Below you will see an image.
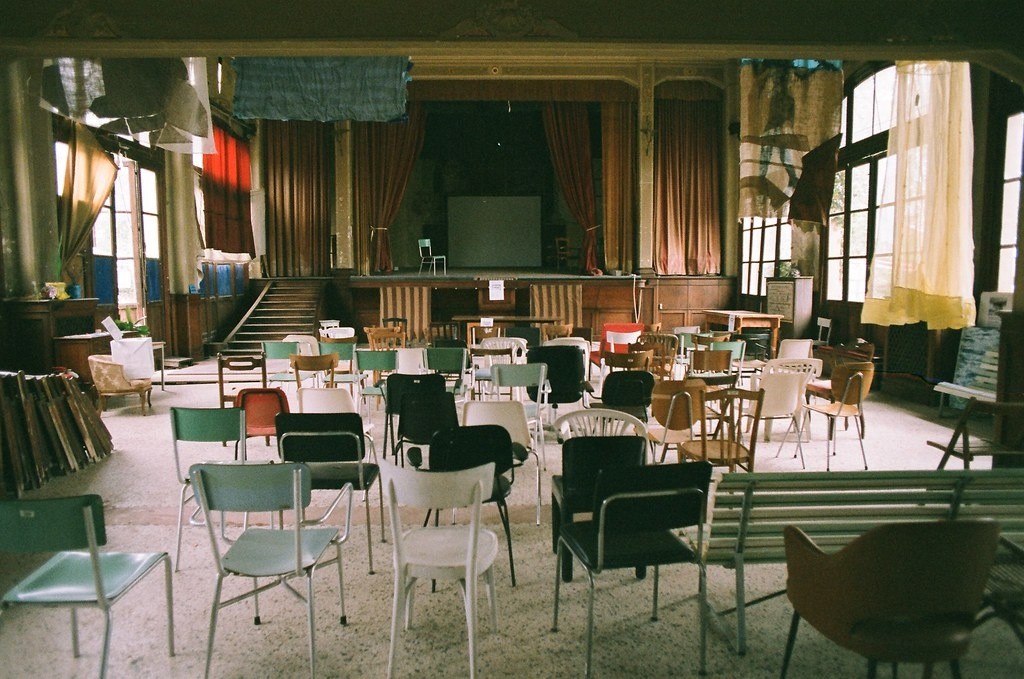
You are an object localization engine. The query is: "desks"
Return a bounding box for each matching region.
[702,309,785,361]
[53,331,140,409]
[149,341,166,392]
[14,299,99,375]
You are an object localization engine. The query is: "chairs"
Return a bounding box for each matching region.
[779,520,1000,678]
[169,407,251,572]
[914,396,1024,508]
[0,493,175,679]
[378,460,498,679]
[676,386,767,510]
[555,238,570,270]
[550,435,655,582]
[217,316,891,586]
[274,413,387,575]
[418,424,516,589]
[88,353,153,416]
[188,462,351,679]
[549,461,718,679]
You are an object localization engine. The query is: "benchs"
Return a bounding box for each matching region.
[702,469,1023,658]
[934,349,1000,428]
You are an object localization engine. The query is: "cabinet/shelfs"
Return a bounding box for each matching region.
[417,239,447,276]
[764,275,815,339]
